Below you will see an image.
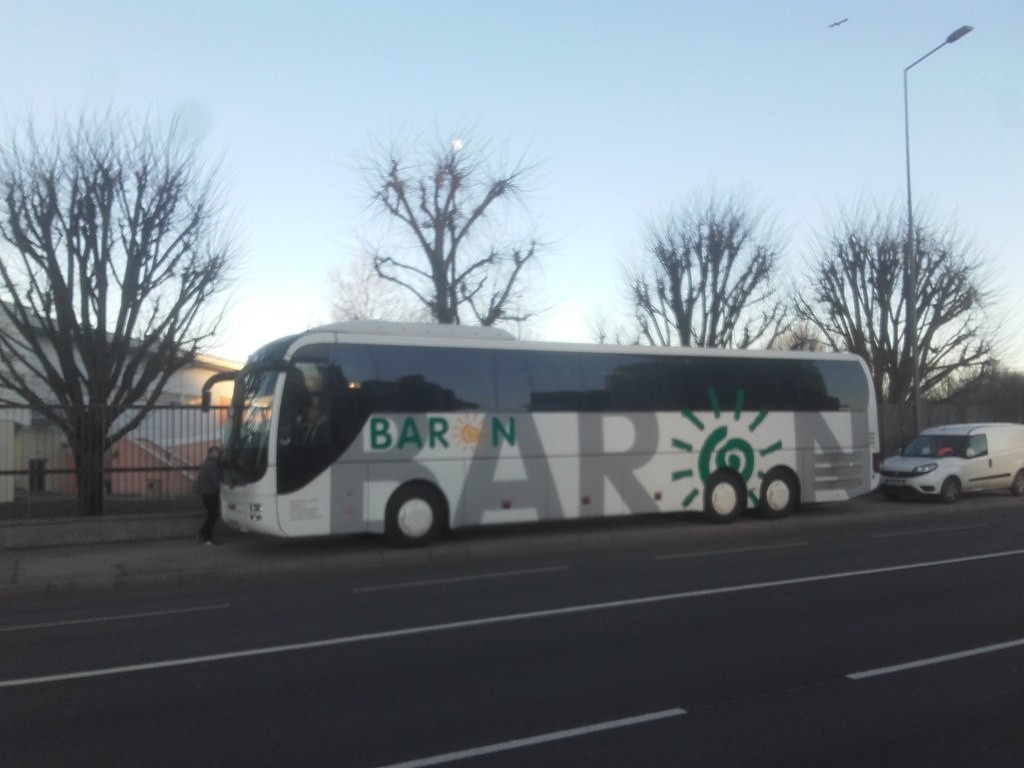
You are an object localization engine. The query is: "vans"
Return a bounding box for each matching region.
[875,420,1023,502]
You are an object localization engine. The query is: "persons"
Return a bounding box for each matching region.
[197,446,223,546]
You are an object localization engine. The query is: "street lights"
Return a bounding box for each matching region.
[901,25,975,438]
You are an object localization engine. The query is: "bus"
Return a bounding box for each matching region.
[201,318,886,551]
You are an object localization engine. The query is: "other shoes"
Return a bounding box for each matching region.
[198,538,220,546]
